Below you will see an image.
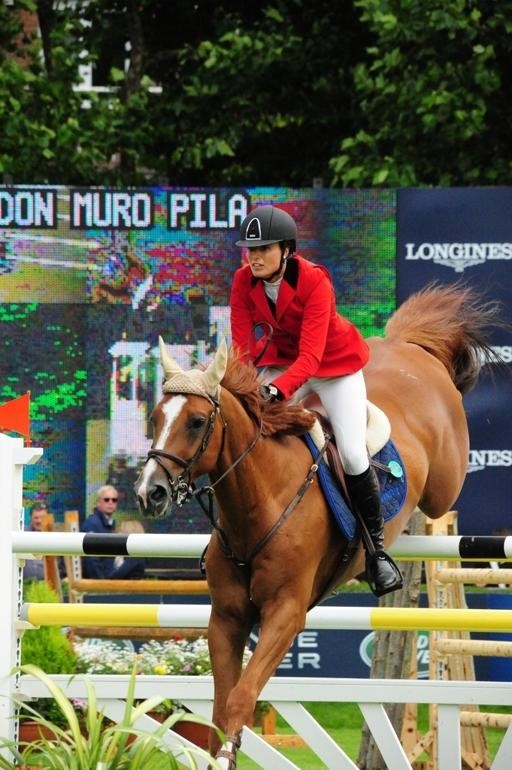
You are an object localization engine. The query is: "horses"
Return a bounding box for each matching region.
[132,272,512,769]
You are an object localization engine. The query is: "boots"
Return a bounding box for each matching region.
[344,467,398,593]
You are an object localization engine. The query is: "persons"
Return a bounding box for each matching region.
[229,205,403,597]
[79,484,124,579]
[23,502,67,581]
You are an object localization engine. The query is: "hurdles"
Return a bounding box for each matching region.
[13,528,511,767]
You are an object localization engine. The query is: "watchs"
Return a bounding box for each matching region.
[269,385,278,397]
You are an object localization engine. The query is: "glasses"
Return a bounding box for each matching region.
[104,498,118,502]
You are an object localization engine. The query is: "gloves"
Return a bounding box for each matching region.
[259,383,280,406]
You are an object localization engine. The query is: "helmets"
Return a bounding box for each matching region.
[235,205,296,248]
